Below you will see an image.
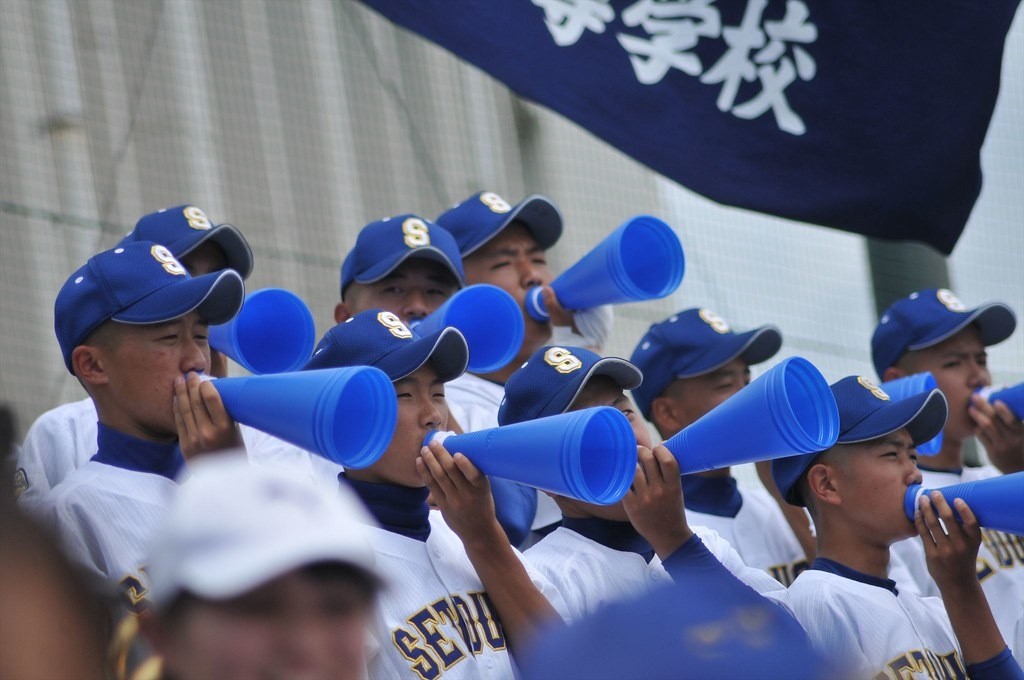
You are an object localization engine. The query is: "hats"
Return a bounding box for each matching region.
[630,308,783,421]
[770,375,949,507]
[498,345,643,427]
[872,287,1017,384]
[302,308,469,384]
[528,580,826,680]
[435,191,562,260]
[340,213,467,303]
[53,240,244,377]
[145,460,398,607]
[117,204,253,280]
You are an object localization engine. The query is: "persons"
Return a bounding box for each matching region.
[0,189,1023,680]
